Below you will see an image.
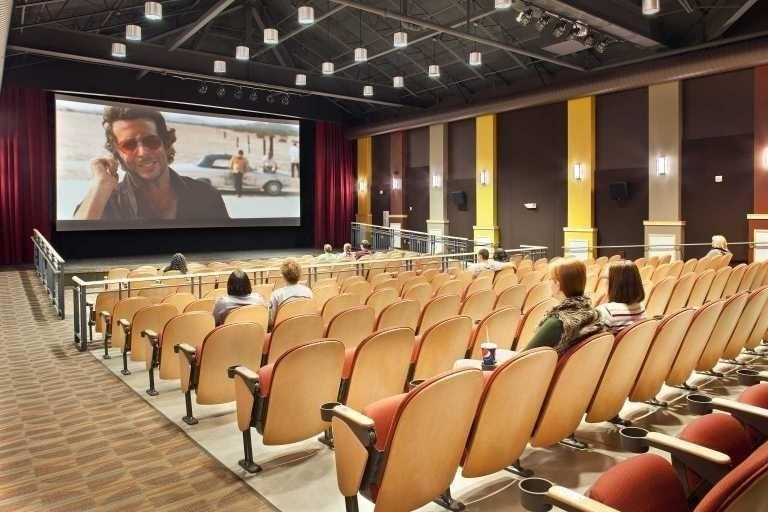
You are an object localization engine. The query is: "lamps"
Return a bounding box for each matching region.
[641,0,659,15]
[495,0,512,8]
[515,0,624,56]
[213,0,482,96]
[110,0,164,57]
[198,79,293,106]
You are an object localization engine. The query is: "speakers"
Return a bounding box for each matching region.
[609,182,628,202]
[451,191,466,205]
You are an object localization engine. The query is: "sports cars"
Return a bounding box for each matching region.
[168,153,289,194]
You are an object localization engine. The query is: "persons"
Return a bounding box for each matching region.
[356,239,372,259]
[268,257,314,328]
[464,248,494,277]
[705,234,730,258]
[213,268,269,326]
[74,105,230,220]
[316,244,338,263]
[261,156,278,174]
[594,259,647,336]
[453,257,605,371]
[491,247,517,273]
[288,142,300,177]
[338,242,354,258]
[230,150,246,197]
[163,252,188,274]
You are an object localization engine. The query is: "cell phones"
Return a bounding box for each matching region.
[109,150,120,177]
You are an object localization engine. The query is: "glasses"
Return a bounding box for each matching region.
[117,135,161,153]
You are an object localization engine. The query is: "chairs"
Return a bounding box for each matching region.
[227,339,344,470]
[618,412,767,498]
[316,367,483,512]
[319,327,417,449]
[172,322,265,424]
[517,442,766,511]
[96,250,768,387]
[685,380,767,422]
[584,316,661,430]
[409,346,561,479]
[523,332,613,451]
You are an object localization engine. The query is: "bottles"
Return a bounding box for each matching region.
[480,343,497,366]
